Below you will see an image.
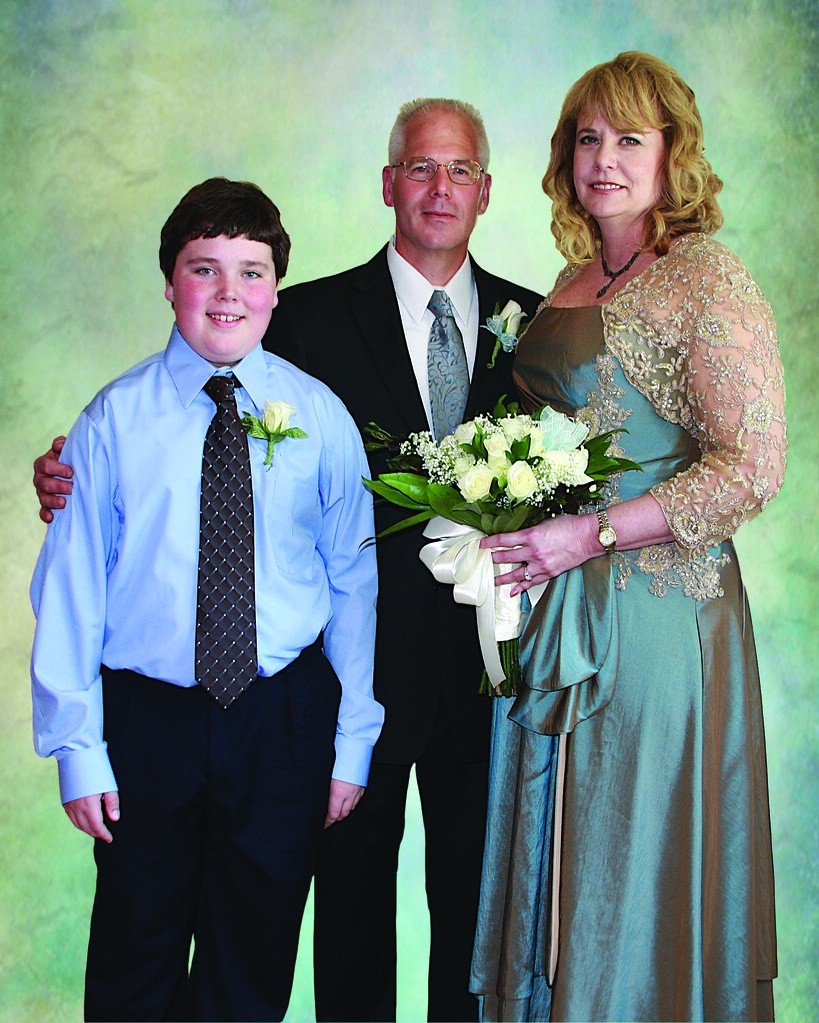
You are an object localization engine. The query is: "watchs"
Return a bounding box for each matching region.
[597,509,617,555]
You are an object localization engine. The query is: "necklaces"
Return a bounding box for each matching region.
[596,241,641,299]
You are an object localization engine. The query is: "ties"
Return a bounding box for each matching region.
[427,291,470,447]
[196,374,258,711]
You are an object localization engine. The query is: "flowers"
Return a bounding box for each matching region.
[356,393,644,699]
[240,400,308,465]
[480,300,528,369]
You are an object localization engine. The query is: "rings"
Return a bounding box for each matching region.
[523,565,533,581]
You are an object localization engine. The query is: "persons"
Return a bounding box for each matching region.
[32,97,546,1023]
[29,176,385,1023]
[466,50,790,1023]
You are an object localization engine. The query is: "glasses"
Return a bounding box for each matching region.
[390,157,485,186]
